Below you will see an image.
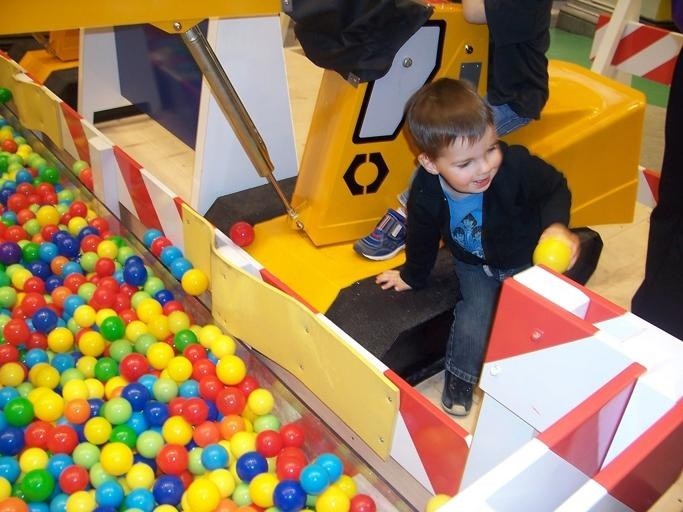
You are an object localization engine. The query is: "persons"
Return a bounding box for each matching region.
[374,78,580,417]
[352,1,550,260]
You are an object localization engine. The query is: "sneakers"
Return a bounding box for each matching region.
[354,209,408,260]
[442,369,473,415]
